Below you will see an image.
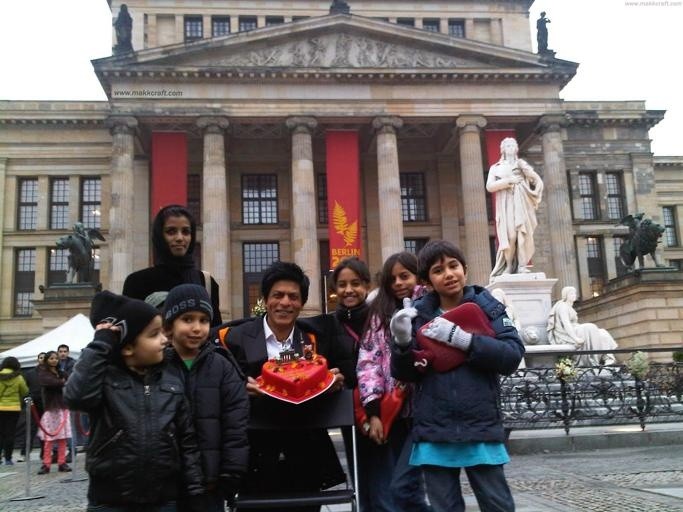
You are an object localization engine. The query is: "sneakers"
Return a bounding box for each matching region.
[16,454,25,461]
[37,465,48,474]
[66,452,70,462]
[58,463,71,471]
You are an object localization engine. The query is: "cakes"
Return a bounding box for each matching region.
[256,344,334,403]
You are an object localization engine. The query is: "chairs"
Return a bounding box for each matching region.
[222,384,359,512]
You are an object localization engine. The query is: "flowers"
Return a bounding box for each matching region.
[552,355,579,384]
[623,350,650,378]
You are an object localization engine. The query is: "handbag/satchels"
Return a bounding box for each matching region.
[418,301,496,373]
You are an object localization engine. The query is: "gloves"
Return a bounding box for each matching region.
[389,297,418,348]
[421,316,473,352]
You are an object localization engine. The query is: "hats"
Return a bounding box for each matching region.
[161,283,214,329]
[90,290,161,362]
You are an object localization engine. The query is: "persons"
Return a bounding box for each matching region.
[352,250,427,511]
[120,203,223,347]
[484,135,543,276]
[490,287,539,378]
[111,3,132,52]
[546,285,619,373]
[536,11,551,54]
[159,283,251,511]
[0,344,78,475]
[206,260,351,511]
[388,238,525,512]
[59,289,206,511]
[305,255,374,512]
